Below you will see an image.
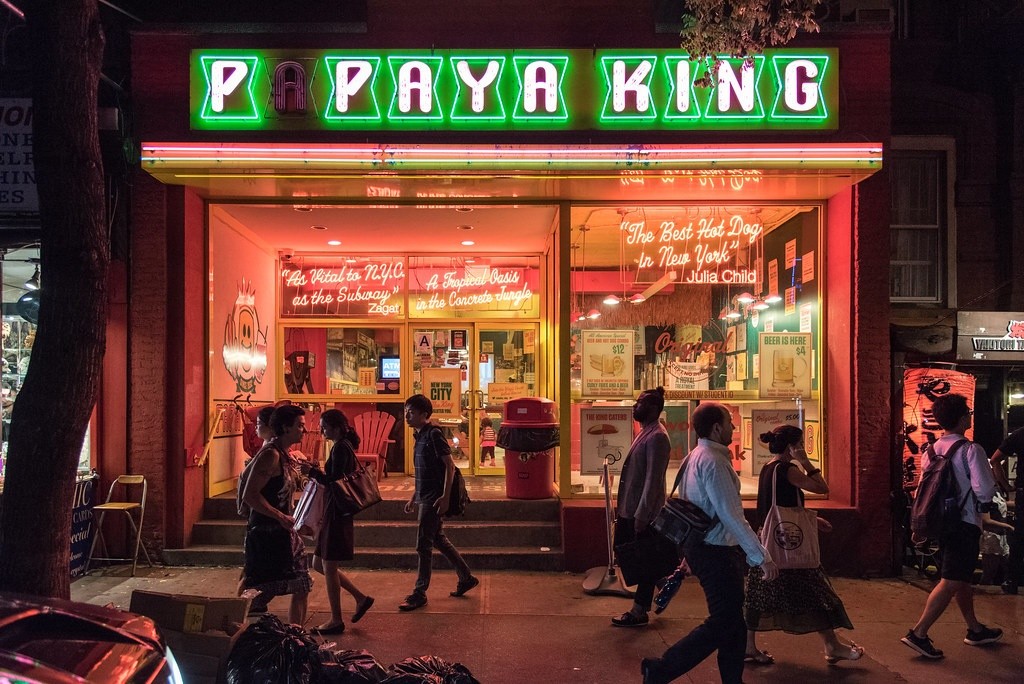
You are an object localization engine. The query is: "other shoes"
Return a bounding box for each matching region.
[351,597,374,623]
[309,623,345,634]
[1001,580,1018,595]
[490,463,496,466]
[480,463,483,466]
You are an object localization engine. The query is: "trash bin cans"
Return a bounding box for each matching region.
[501,397,560,500]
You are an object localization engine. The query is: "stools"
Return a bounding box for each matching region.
[84,475,154,578]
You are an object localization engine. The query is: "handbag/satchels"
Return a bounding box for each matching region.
[332,441,382,515]
[430,427,471,516]
[761,464,821,568]
[648,456,719,561]
[238,444,269,519]
[291,478,330,541]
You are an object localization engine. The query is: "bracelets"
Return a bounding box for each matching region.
[807,468,822,478]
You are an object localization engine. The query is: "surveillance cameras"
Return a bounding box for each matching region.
[284,248,294,260]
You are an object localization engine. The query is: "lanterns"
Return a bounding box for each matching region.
[902,366,975,497]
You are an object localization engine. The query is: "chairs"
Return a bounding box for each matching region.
[348,412,397,481]
[288,411,322,461]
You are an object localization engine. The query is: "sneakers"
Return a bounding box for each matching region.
[900,629,944,658]
[654,601,669,615]
[399,592,428,611]
[964,624,1003,646]
[641,657,659,684]
[450,574,479,597]
[611,612,649,627]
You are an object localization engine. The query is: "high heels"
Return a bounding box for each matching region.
[744,649,774,666]
[825,642,865,664]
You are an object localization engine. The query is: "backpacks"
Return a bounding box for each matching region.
[910,439,973,540]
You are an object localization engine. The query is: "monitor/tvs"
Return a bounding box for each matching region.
[378,355,400,382]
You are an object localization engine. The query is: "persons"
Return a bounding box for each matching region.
[237,405,313,628]
[991,425,1024,592]
[980,457,1015,558]
[461,401,496,466]
[638,402,779,684]
[611,387,690,627]
[744,423,865,664]
[900,393,1004,657]
[297,409,376,634]
[398,393,480,611]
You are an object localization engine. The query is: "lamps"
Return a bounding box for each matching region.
[572,226,677,322]
[717,211,781,320]
